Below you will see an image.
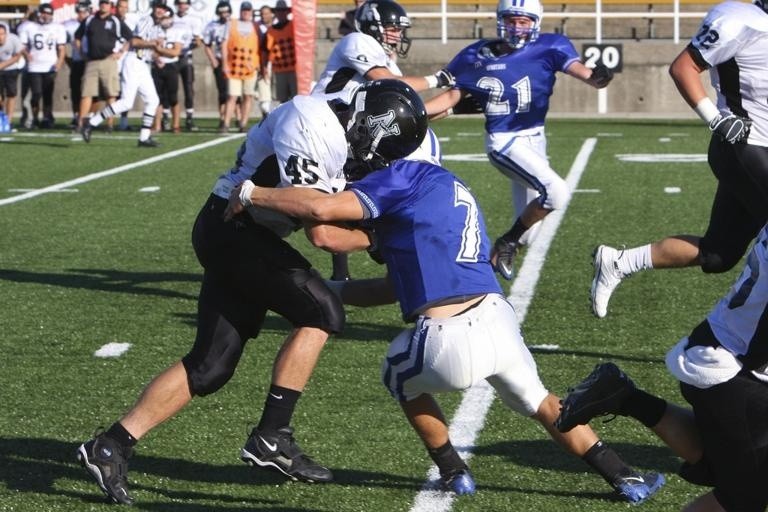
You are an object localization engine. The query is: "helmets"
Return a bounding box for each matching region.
[493,0,544,50]
[75,2,90,13]
[331,77,427,181]
[216,1,231,15]
[354,0,413,59]
[36,2,53,23]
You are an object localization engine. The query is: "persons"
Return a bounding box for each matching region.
[423,0,614,284]
[223,126,665,505]
[590,0,766,321]
[308,0,484,282]
[337,1,366,37]
[551,227,766,511]
[73,77,431,510]
[1,0,300,147]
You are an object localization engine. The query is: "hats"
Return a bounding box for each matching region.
[240,1,251,10]
[268,0,291,15]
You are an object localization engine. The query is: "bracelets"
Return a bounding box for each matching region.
[695,96,720,125]
[425,74,439,90]
[240,178,255,207]
[323,279,349,306]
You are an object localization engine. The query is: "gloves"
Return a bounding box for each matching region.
[434,67,457,90]
[708,110,752,146]
[452,94,482,114]
[589,63,615,88]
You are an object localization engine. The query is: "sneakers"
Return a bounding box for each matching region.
[436,469,474,495]
[185,118,198,132]
[493,235,516,282]
[615,473,665,507]
[74,425,135,506]
[81,117,92,143]
[553,362,634,435]
[588,243,625,318]
[237,421,333,484]
[138,136,161,146]
